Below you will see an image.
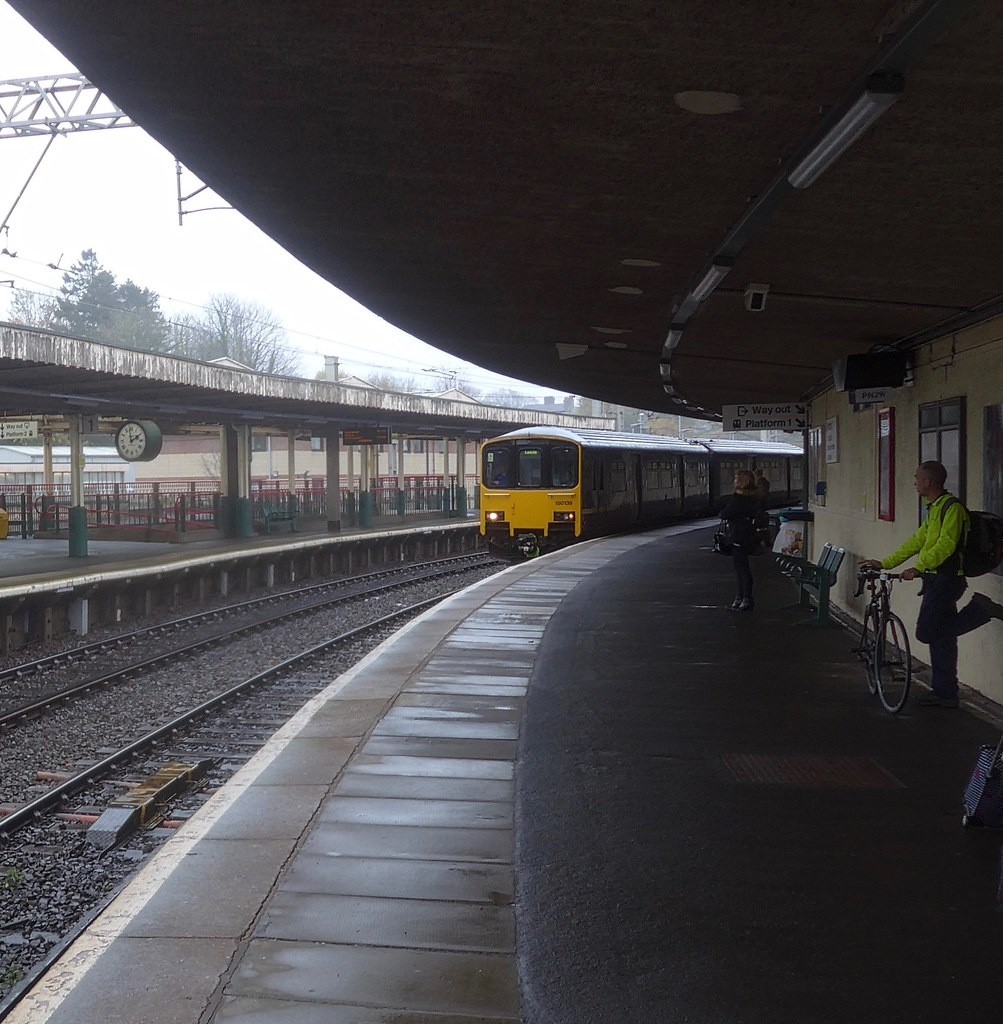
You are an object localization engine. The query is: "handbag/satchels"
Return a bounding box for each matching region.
[712,519,736,556]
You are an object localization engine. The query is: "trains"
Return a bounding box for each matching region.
[479,427,805,566]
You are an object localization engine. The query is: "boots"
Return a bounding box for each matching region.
[731,581,752,610]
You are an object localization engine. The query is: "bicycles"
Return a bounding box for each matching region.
[852,566,938,715]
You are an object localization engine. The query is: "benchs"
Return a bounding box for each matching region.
[262,506,296,535]
[775,542,845,623]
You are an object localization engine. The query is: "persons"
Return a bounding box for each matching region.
[491,460,509,485]
[718,469,770,613]
[858,461,1003,708]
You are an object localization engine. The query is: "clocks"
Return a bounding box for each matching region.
[114,419,163,462]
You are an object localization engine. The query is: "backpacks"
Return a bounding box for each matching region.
[941,497,1003,577]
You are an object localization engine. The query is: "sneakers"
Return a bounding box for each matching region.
[972,593,1003,621]
[911,693,960,708]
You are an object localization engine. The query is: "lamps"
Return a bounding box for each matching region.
[664,384,674,394]
[663,325,684,350]
[659,364,671,376]
[787,79,905,189]
[688,259,733,302]
